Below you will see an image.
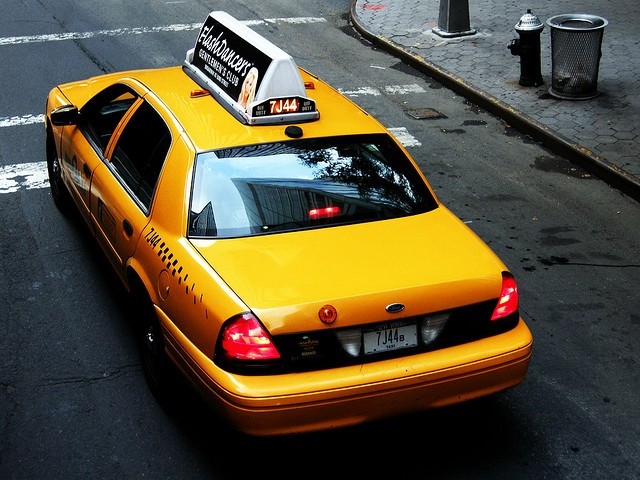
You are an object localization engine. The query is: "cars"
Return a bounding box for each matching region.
[44,11,534,436]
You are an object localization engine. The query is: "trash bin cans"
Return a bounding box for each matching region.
[545,13,609,99]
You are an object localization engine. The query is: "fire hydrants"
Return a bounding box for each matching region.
[508,9,545,86]
